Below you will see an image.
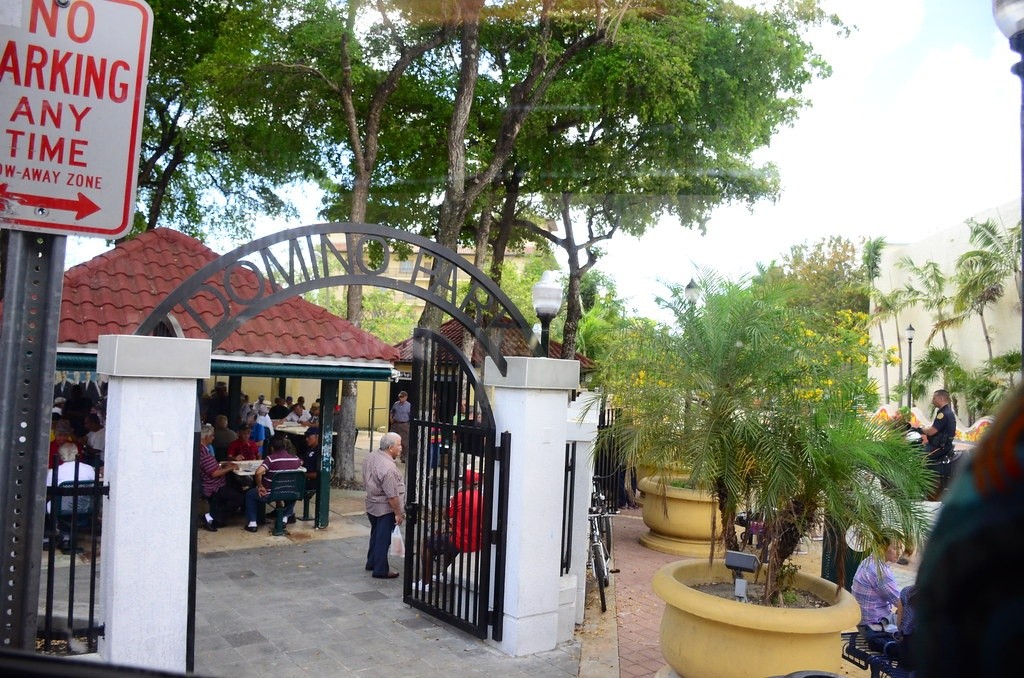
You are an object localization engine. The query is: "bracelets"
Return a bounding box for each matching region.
[918,426,923,434]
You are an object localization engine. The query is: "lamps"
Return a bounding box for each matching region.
[530,269,563,358]
[726,550,760,598]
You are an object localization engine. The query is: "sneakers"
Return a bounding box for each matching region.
[432,573,450,584]
[412,579,429,593]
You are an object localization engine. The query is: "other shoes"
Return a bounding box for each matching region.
[275,522,286,529]
[200,512,217,531]
[365,564,399,579]
[288,513,296,524]
[245,524,257,532]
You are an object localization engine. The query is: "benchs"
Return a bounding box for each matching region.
[838,631,912,678]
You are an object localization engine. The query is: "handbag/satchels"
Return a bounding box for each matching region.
[391,525,405,558]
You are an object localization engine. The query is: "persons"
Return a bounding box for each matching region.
[431,398,486,470]
[198,381,320,532]
[412,470,484,592]
[43,384,108,550]
[389,391,411,463]
[362,432,405,579]
[54,370,109,398]
[849,382,1024,678]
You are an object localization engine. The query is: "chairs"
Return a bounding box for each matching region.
[256,471,305,537]
[46,480,103,556]
[298,456,337,521]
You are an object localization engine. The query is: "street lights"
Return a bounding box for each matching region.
[685,278,700,437]
[905,323,916,409]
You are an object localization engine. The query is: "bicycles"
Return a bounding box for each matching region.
[591,474,620,612]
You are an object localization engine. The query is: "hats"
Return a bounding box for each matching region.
[466,470,479,484]
[304,427,319,435]
[398,391,408,397]
[239,423,251,430]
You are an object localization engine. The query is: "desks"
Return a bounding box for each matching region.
[220,460,307,477]
[271,419,338,436]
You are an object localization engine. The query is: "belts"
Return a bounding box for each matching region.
[394,420,405,424]
